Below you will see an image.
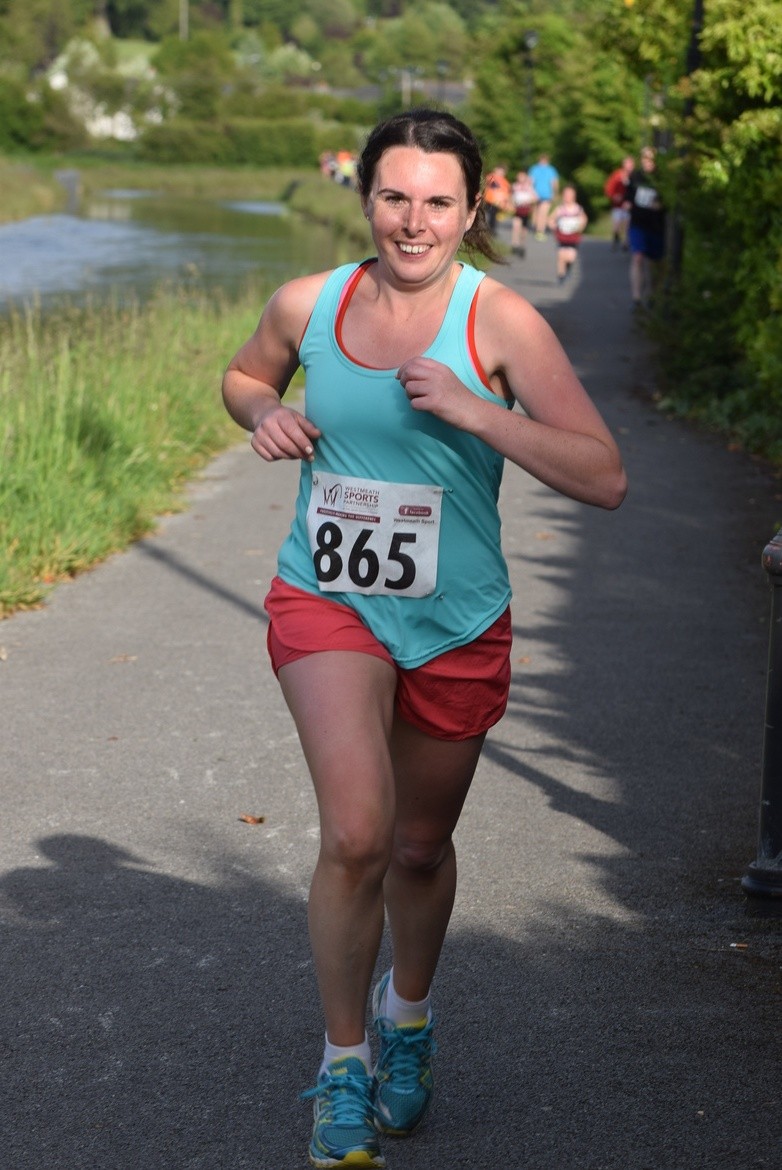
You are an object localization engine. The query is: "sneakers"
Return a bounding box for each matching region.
[372,969,440,1134]
[298,1053,386,1170]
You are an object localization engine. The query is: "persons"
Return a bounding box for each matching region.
[622,145,668,315]
[511,172,537,258]
[547,187,588,285]
[483,166,512,230]
[221,108,626,1170]
[605,157,635,251]
[528,153,559,241]
[317,148,354,186]
